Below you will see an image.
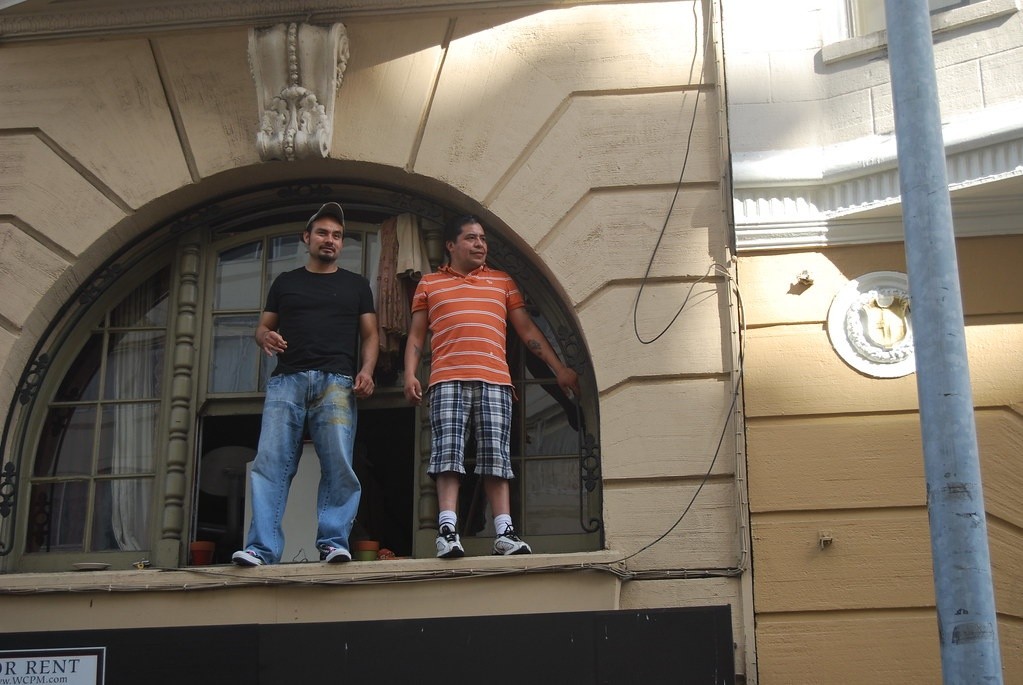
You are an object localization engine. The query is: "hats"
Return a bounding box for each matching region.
[306,202,345,236]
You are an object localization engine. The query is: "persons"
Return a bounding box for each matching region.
[403,214,580,558]
[232,202,379,566]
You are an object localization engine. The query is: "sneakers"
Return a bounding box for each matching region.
[318,544,351,563]
[232,549,265,566]
[433,525,465,558]
[491,522,532,555]
